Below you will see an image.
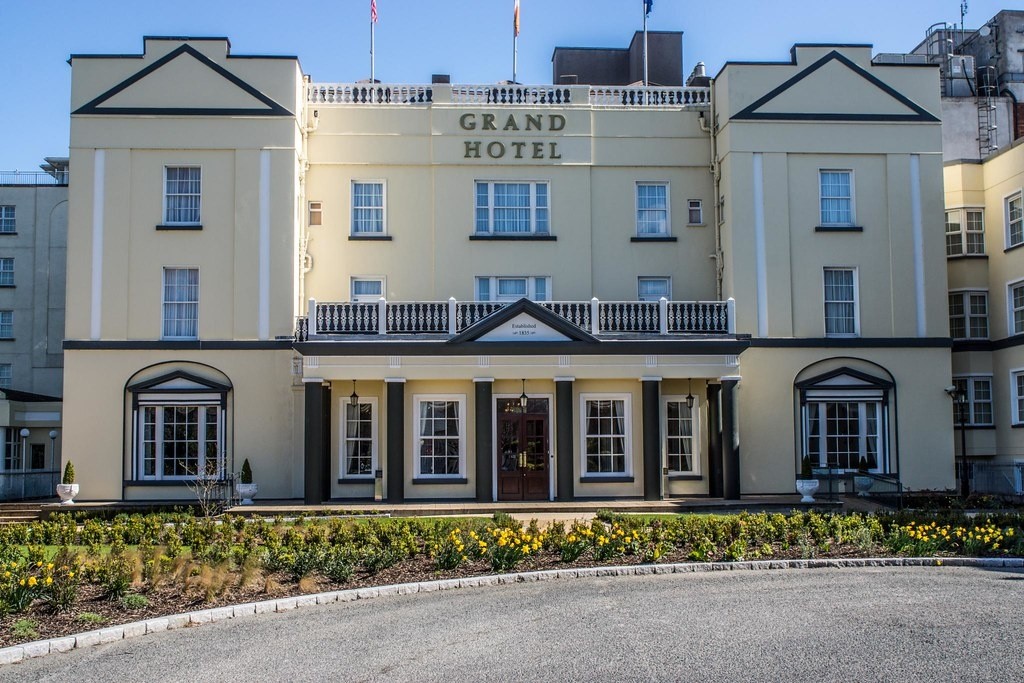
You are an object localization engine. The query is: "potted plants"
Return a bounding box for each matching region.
[854,456,874,497]
[56,460,80,505]
[796,455,820,502]
[236,459,258,506]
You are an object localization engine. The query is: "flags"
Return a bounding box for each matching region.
[513,0,521,38]
[371,0,378,23]
[643,0,653,18]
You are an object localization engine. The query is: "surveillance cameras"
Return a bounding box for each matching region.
[944,386,956,392]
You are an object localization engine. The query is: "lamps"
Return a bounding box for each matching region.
[519,379,528,407]
[686,378,694,410]
[20,429,30,437]
[49,431,57,439]
[350,379,359,408]
